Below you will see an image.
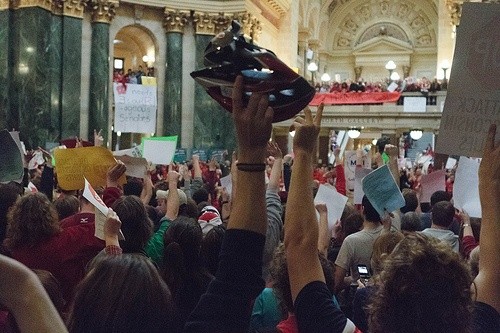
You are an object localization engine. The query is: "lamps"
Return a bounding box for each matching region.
[306,62,331,86]
[441,60,449,82]
[347,128,362,139]
[386,59,400,83]
[409,129,423,141]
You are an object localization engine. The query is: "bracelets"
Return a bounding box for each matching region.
[236,162,266,171]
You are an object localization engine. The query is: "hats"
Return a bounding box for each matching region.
[197,205,222,240]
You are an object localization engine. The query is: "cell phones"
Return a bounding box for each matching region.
[356,264,372,286]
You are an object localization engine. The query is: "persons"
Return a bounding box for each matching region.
[403,142,408,157]
[0,74,500,333]
[113,65,154,84]
[314,77,447,106]
